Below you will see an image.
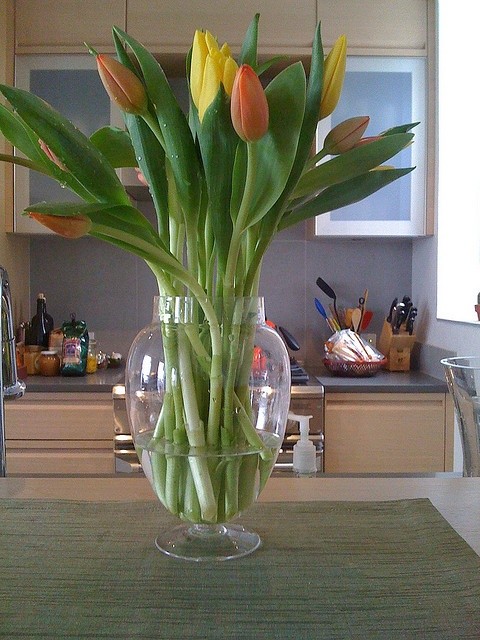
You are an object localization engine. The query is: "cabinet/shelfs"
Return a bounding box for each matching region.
[1,55,122,235]
[324,391,454,473]
[2,390,115,473]
[313,56,438,237]
[127,1,318,56]
[0,0,128,55]
[317,2,440,55]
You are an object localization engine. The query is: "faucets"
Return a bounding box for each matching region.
[1,265,28,478]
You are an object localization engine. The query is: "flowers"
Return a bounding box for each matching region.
[0,13,420,295]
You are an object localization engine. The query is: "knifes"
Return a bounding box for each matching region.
[387,295,417,336]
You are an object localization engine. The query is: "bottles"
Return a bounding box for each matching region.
[30,293,54,348]
[15,340,25,367]
[23,345,44,376]
[85,331,98,374]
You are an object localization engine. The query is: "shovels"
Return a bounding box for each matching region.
[313,297,336,331]
[352,308,361,332]
[316,276,343,330]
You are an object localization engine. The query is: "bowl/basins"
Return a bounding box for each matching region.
[108,356,121,369]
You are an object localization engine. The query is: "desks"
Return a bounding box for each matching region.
[1,479,478,639]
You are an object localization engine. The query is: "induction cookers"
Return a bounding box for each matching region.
[290,356,311,384]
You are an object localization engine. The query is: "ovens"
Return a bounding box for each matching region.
[107,375,325,477]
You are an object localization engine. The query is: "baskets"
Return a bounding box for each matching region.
[321,356,388,377]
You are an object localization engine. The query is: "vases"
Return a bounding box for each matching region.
[122,295,292,566]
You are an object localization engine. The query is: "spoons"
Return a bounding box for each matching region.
[350,308,362,333]
[313,298,328,321]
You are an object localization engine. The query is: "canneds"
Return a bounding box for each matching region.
[24,344,40,375]
